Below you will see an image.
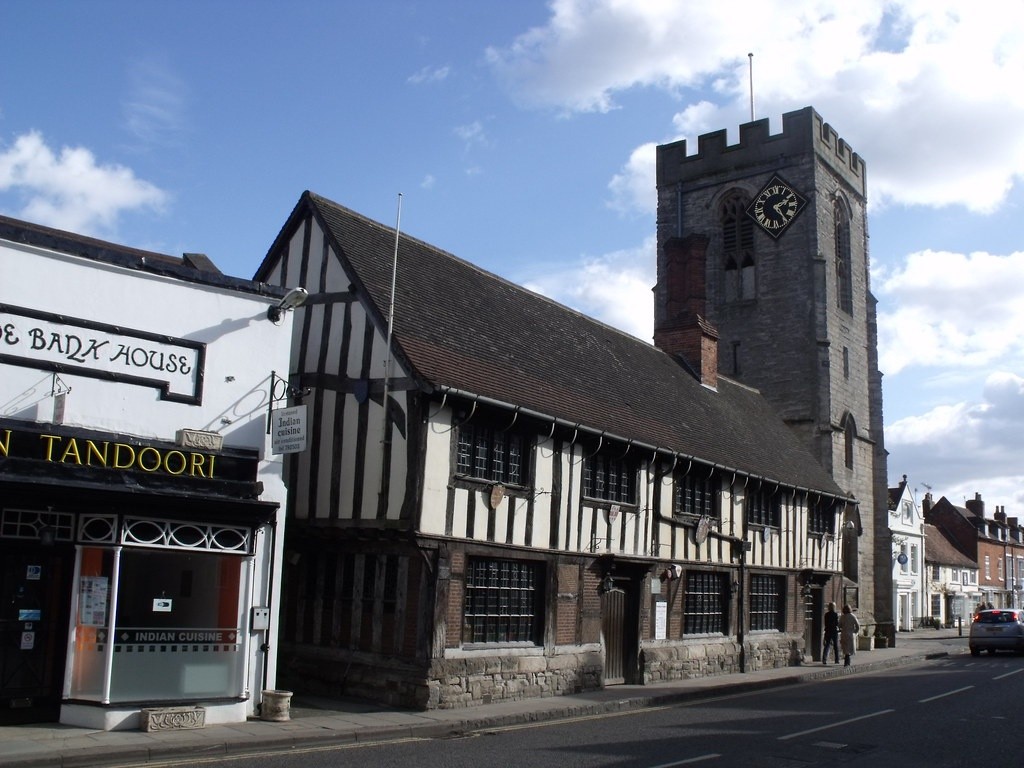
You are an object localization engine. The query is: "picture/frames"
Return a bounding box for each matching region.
[845,586,860,611]
[963,572,968,586]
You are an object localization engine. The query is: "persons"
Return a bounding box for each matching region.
[822,602,841,665]
[980,601,988,611]
[988,603,994,609]
[838,603,860,667]
[974,602,981,614]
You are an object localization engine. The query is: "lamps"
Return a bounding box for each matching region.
[843,521,855,530]
[729,580,739,600]
[802,580,812,595]
[602,572,614,591]
[267,287,309,327]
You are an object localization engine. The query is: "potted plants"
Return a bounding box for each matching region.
[176,428,223,451]
[946,617,955,628]
[859,623,875,650]
[876,632,889,648]
[933,618,941,630]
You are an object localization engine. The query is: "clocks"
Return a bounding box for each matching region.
[743,172,811,241]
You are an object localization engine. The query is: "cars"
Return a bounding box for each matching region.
[967,608,1024,656]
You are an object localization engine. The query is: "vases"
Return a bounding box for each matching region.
[140,707,206,732]
